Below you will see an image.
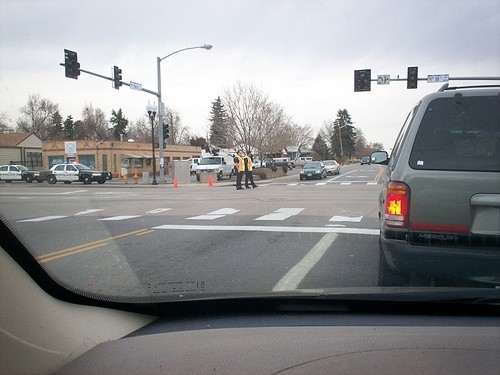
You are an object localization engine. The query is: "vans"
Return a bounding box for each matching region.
[270,158,290,166]
[187,158,202,175]
[196,156,235,181]
[294,156,313,166]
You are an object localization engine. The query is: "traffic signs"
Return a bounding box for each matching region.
[427,75,449,84]
[129,80,148,92]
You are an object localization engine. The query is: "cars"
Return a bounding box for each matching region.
[322,160,340,176]
[39,161,112,184]
[252,157,269,168]
[300,161,327,179]
[0,164,40,183]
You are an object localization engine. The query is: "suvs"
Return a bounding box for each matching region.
[368,82,500,285]
[361,157,370,165]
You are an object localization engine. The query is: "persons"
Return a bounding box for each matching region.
[234,151,245,189]
[245,152,258,188]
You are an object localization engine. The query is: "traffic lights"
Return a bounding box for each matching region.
[354,69,371,91]
[163,123,170,141]
[114,65,122,90]
[408,66,419,89]
[65,48,80,80]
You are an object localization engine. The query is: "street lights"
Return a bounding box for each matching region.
[147,101,157,185]
[157,44,213,184]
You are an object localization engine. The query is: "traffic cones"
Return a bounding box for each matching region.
[208,174,214,187]
[173,175,178,188]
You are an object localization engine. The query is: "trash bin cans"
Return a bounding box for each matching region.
[142,172,149,184]
